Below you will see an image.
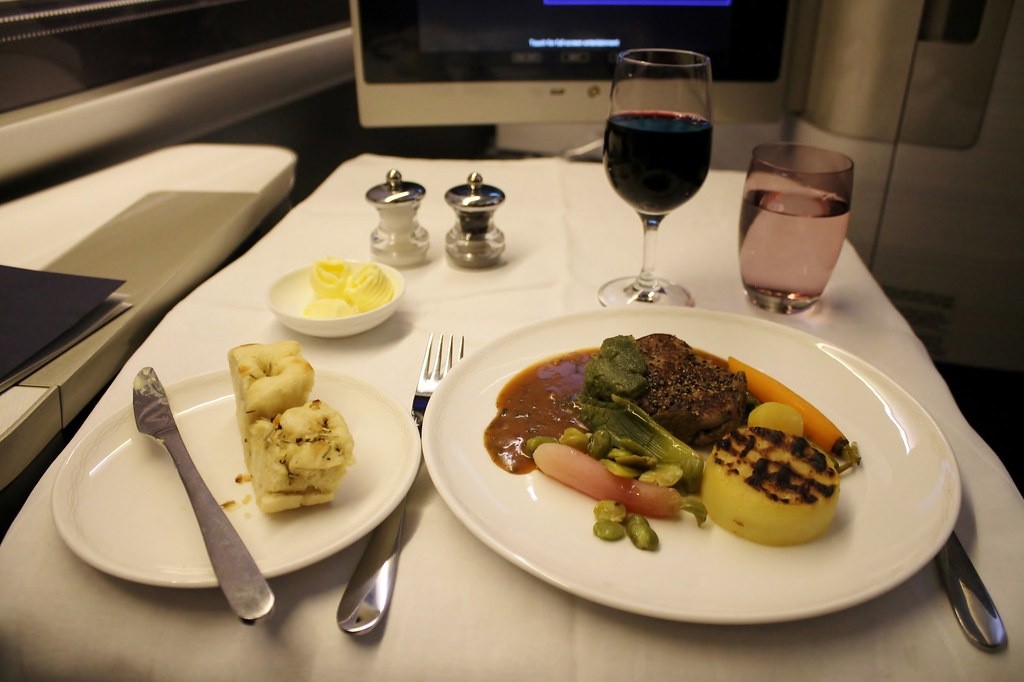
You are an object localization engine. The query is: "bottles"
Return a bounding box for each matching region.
[444,171,506,268]
[365,168,429,266]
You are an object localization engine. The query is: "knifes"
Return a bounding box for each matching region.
[131,366,276,627]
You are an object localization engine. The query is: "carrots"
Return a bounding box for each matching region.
[727,356,862,473]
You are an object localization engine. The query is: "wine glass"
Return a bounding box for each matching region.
[597,49,713,306]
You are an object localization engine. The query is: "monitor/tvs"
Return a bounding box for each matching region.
[350,0,798,162]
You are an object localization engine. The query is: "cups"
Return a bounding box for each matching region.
[738,142,855,314]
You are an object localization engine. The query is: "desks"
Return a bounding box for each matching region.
[0,140,1024,682]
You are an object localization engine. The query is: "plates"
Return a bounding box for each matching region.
[266,259,406,337]
[51,363,421,589]
[421,304,961,625]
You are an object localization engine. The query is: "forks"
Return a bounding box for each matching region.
[337,331,464,635]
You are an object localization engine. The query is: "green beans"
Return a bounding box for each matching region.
[528,428,683,550]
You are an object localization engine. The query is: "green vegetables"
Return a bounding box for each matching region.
[570,394,704,492]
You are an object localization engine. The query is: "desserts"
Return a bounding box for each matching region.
[228,340,355,514]
[303,258,394,319]
[708,426,837,545]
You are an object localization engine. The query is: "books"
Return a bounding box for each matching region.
[0,264,133,394]
